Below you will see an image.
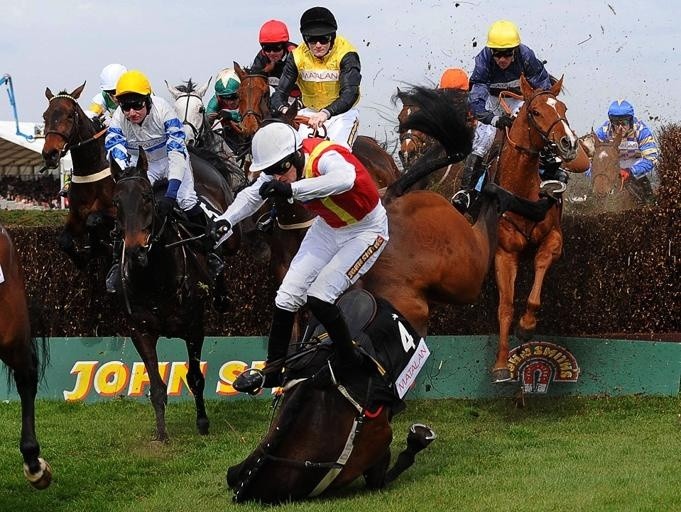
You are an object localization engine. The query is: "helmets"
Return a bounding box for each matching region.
[248,122,303,171]
[214,67,242,96]
[439,68,469,91]
[101,64,151,97]
[300,6,337,35]
[259,18,289,43]
[607,99,634,117]
[485,20,521,48]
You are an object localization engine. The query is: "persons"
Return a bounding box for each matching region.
[438,67,477,131]
[82,62,132,127]
[202,121,393,394]
[449,18,571,212]
[203,67,246,131]
[267,8,363,156]
[583,99,661,202]
[102,70,227,297]
[0,172,70,210]
[248,19,306,119]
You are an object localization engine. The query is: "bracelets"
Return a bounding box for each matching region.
[321,109,332,118]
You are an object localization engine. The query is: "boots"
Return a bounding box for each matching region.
[183,201,225,267]
[105,202,126,294]
[303,293,358,379]
[232,307,297,392]
[452,155,489,209]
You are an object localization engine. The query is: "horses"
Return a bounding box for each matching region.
[109,145,251,444]
[481,73,580,386]
[39,79,240,336]
[585,126,639,215]
[225,78,497,505]
[0,225,53,490]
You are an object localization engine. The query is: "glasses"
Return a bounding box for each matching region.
[611,118,628,125]
[262,43,282,51]
[119,100,146,111]
[493,48,513,57]
[218,94,238,100]
[262,152,295,175]
[306,34,330,44]
